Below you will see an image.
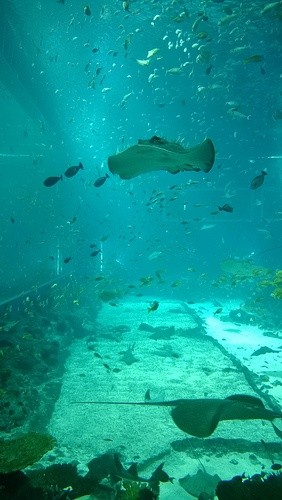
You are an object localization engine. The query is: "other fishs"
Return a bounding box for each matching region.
[1,0,282,500]
